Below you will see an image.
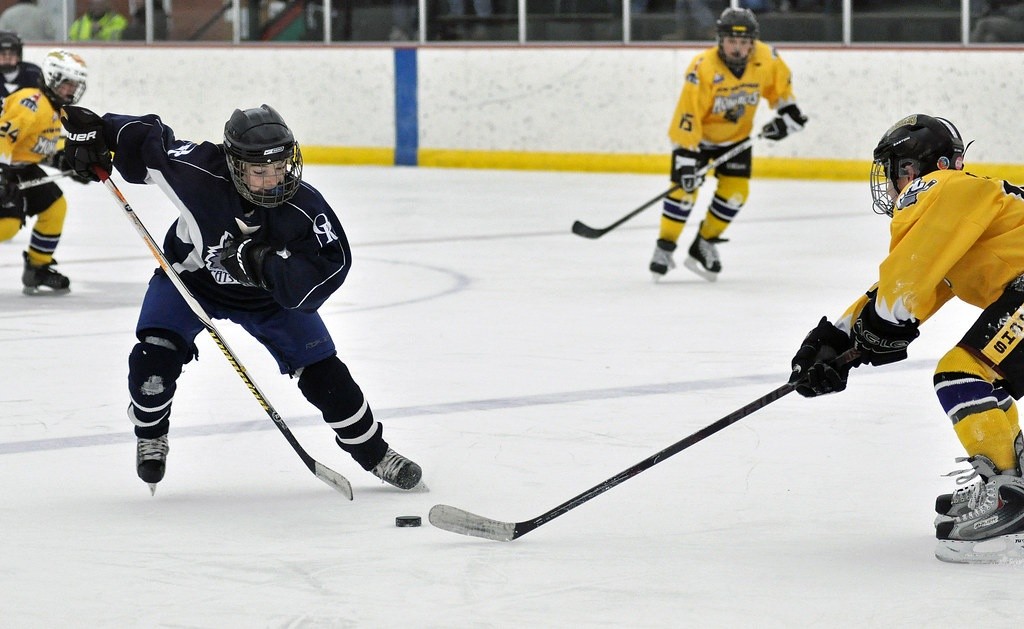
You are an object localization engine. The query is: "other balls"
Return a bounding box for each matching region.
[396,516,421,527]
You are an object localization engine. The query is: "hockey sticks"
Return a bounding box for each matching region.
[572,131,765,239]
[94,165,353,501]
[15,170,79,191]
[428,347,863,542]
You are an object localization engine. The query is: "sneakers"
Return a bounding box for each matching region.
[935,449,1024,563]
[22,251,71,294]
[647,238,677,281]
[684,220,729,283]
[370,447,430,492]
[136,434,169,496]
[934,430,1024,528]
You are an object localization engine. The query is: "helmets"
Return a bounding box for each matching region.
[46,51,88,105]
[716,7,760,37]
[223,104,294,206]
[874,113,964,180]
[0,33,22,73]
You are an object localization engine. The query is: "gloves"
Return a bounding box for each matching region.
[221,238,260,287]
[52,140,112,184]
[850,287,922,368]
[788,316,852,397]
[762,105,804,140]
[672,149,706,194]
[62,104,113,154]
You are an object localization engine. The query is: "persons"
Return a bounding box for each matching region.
[68,0,170,41]
[0,32,48,113]
[970,0,1024,42]
[787,114,1024,564]
[648,9,808,284]
[0,51,114,295]
[59,99,431,498]
[440,0,494,34]
[659,0,719,41]
